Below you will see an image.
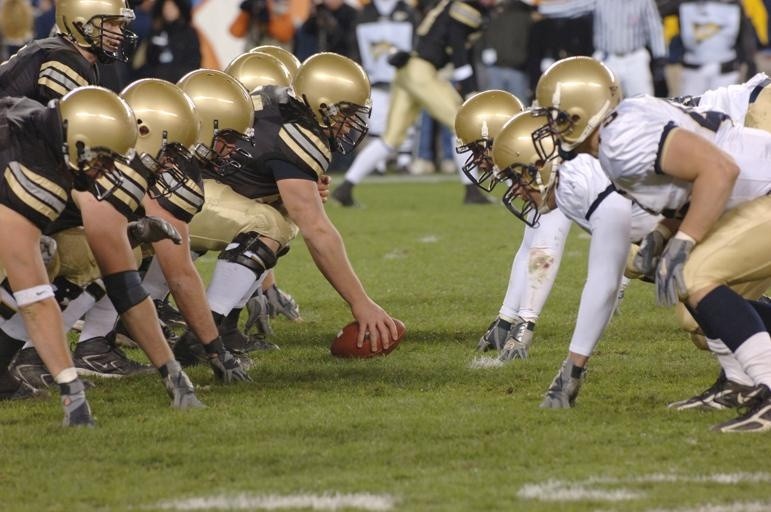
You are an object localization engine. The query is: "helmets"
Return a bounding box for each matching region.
[58,86,138,202]
[176,69,256,180]
[454,90,524,192]
[224,53,293,94]
[491,110,565,229]
[293,50,374,156]
[530,56,624,166]
[54,1,137,63]
[118,79,202,200]
[250,44,301,77]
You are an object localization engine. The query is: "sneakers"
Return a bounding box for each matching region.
[665,367,755,411]
[463,184,496,203]
[12,346,96,396]
[222,309,279,353]
[70,331,157,378]
[331,181,365,208]
[154,298,186,326]
[0,329,52,400]
[173,328,254,370]
[116,311,180,346]
[707,382,771,435]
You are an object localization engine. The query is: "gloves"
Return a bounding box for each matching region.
[654,237,694,307]
[537,359,585,410]
[204,336,254,386]
[498,321,532,362]
[262,282,300,322]
[129,215,183,245]
[244,288,272,336]
[471,316,512,354]
[162,369,209,411]
[58,377,92,427]
[632,229,665,277]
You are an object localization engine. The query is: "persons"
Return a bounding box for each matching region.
[231,0,310,58]
[536,57,771,437]
[1,86,137,429]
[304,0,366,65]
[250,46,301,323]
[10,68,255,394]
[403,108,456,175]
[492,109,771,410]
[680,1,740,97]
[0,0,35,59]
[347,0,423,174]
[128,0,222,86]
[0,0,137,106]
[537,0,669,99]
[455,74,771,364]
[332,0,504,208]
[0,79,212,412]
[224,54,292,338]
[172,52,398,367]
[474,0,563,108]
[31,0,56,41]
[112,0,156,90]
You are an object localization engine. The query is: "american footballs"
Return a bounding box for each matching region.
[330,316,407,359]
